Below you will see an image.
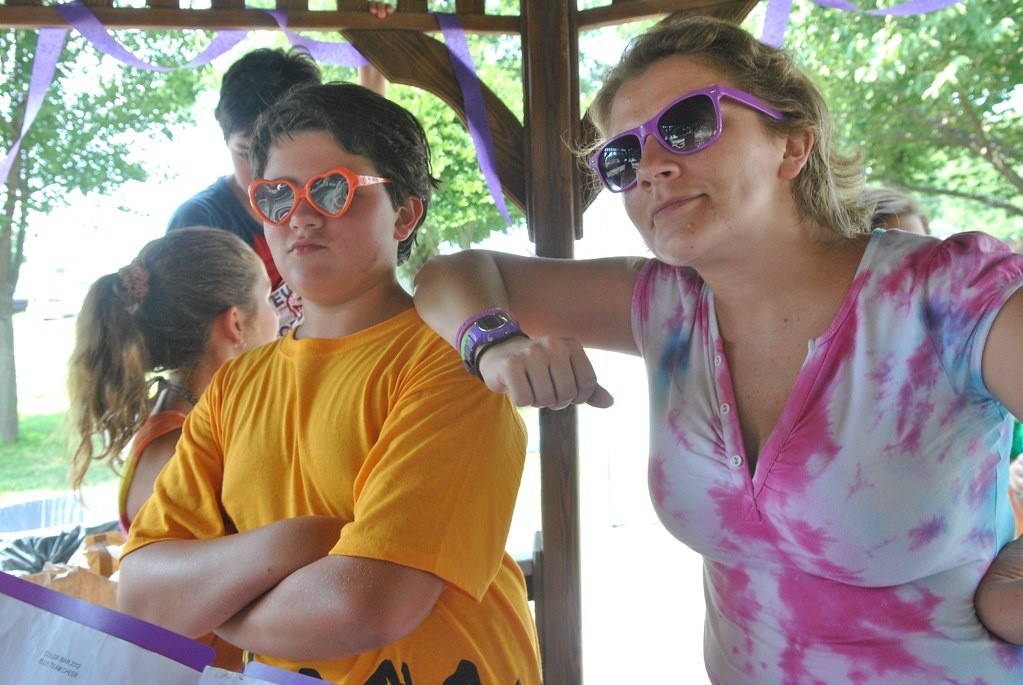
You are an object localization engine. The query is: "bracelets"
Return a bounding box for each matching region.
[454,305,519,356]
[473,333,530,385]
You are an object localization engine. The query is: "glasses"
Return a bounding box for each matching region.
[248,168,394,227]
[590,84,785,193]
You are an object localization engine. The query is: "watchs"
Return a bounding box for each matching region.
[463,314,523,376]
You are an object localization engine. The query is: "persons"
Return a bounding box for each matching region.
[167,43,322,338]
[117,81,544,685]
[410,17,1023,685]
[68,225,281,538]
